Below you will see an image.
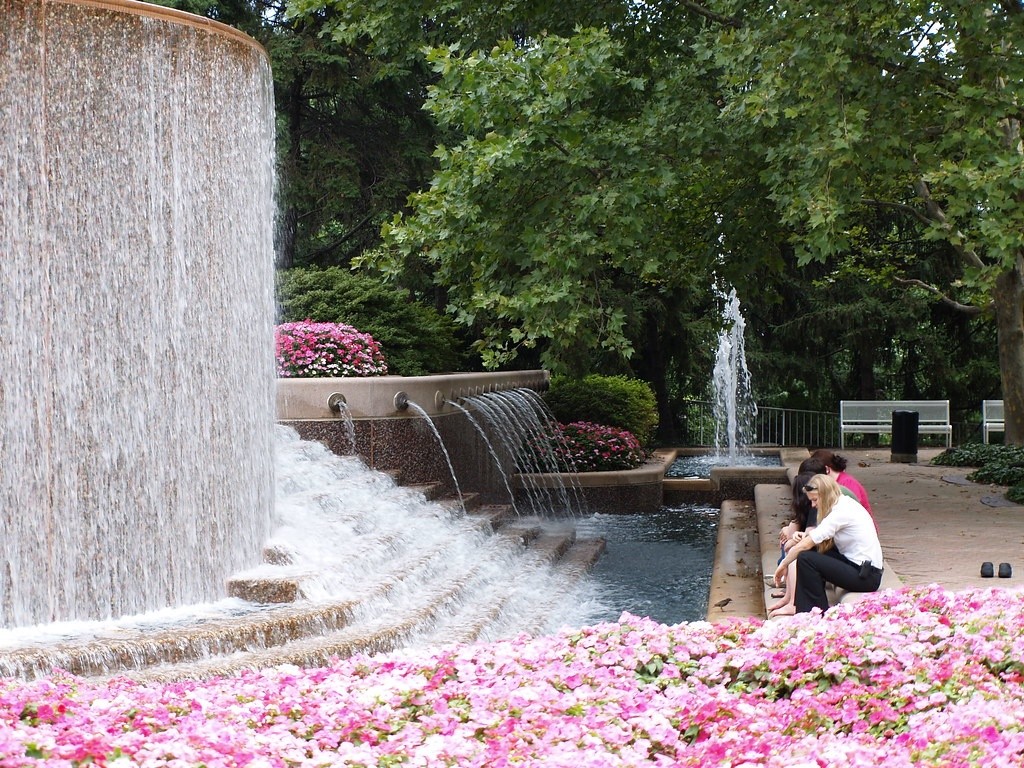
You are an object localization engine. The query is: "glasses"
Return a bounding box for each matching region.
[802,482,818,492]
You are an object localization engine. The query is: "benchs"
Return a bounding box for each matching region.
[983,399,1005,443]
[840,398,952,449]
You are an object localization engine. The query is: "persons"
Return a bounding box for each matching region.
[764,471,816,619]
[794,474,884,617]
[764,460,859,597]
[812,449,877,536]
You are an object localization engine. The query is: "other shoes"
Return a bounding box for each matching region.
[999,563,1012,578]
[765,575,786,588]
[980,562,994,578]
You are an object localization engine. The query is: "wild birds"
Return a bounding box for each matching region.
[713,598,733,612]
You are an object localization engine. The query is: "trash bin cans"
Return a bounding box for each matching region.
[889,409,920,464]
